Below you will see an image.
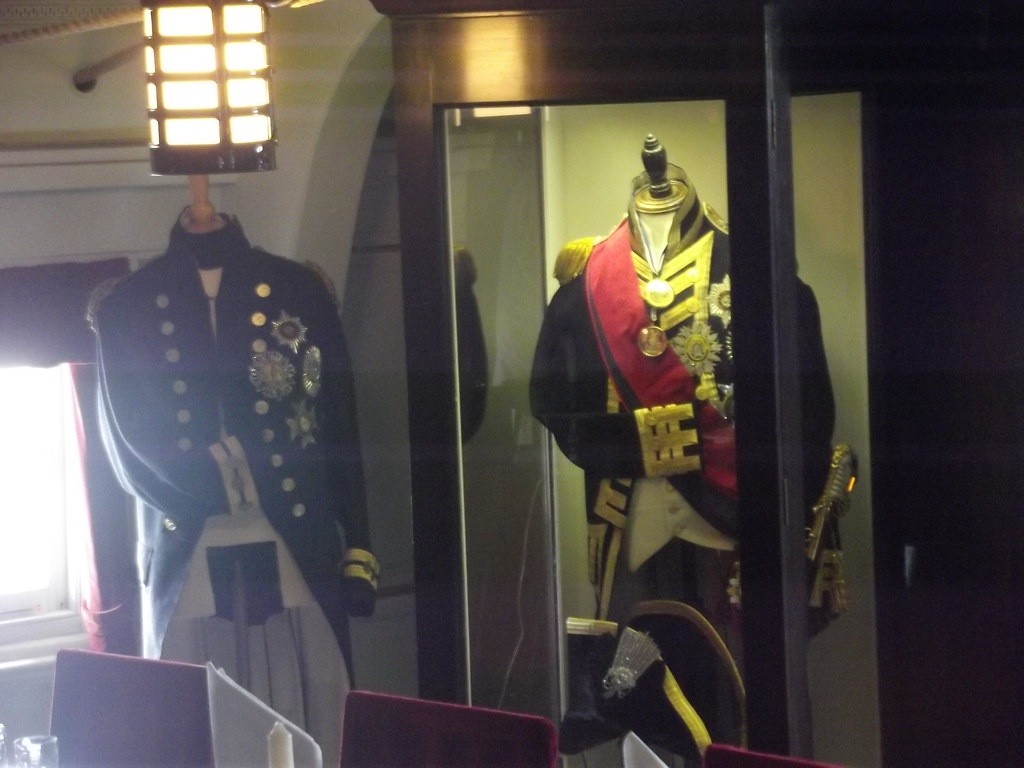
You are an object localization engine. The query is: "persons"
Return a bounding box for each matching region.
[528,133,837,750]
[91,173,380,768]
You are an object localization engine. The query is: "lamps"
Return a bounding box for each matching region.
[137,1,278,176]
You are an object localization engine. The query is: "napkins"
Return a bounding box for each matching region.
[207,660,322,768]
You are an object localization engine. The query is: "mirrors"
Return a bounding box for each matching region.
[444,92,556,768]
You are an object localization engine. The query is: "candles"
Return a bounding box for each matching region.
[268,721,295,767]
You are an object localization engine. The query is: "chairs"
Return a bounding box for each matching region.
[48,649,214,768]
[340,690,558,768]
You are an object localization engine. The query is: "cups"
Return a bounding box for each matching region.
[0,724,9,768]
[14,736,59,768]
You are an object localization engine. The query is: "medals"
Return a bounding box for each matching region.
[637,326,667,357]
[643,279,675,309]
